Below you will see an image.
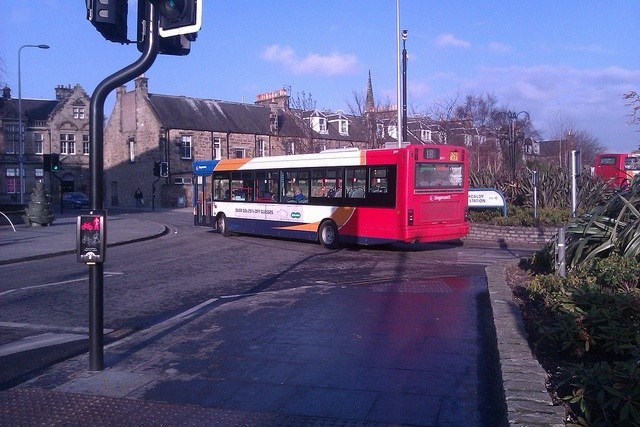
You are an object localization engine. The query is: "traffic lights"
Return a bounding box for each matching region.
[153,161,160,176]
[162,162,167,175]
[52,153,60,171]
[43,154,51,172]
[159,0,202,37]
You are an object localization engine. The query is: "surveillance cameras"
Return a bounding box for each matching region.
[403,29,409,42]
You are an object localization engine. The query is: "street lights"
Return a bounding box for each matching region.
[494,111,530,202]
[18,45,50,205]
[401,29,408,142]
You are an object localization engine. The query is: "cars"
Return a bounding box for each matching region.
[62,191,90,209]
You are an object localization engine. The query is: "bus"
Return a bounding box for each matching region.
[191,144,470,250]
[594,153,640,192]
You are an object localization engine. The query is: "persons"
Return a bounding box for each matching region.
[282,187,305,203]
